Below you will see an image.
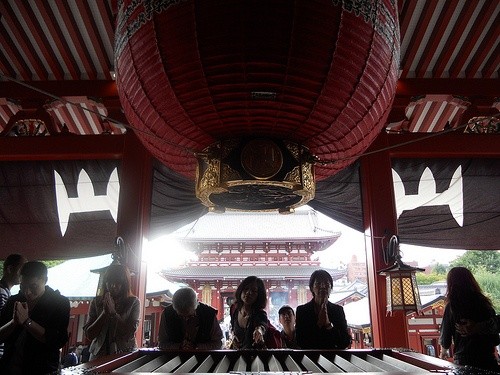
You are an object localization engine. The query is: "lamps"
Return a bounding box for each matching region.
[89,237,136,295]
[378,234,425,318]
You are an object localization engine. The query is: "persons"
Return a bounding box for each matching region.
[0,254,351,375]
[438,267,500,372]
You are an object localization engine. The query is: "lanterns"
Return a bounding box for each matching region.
[114,0,400,215]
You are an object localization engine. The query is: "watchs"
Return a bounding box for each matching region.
[326,322,333,330]
[22,318,32,328]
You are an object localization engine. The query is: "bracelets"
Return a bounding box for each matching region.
[109,312,119,317]
